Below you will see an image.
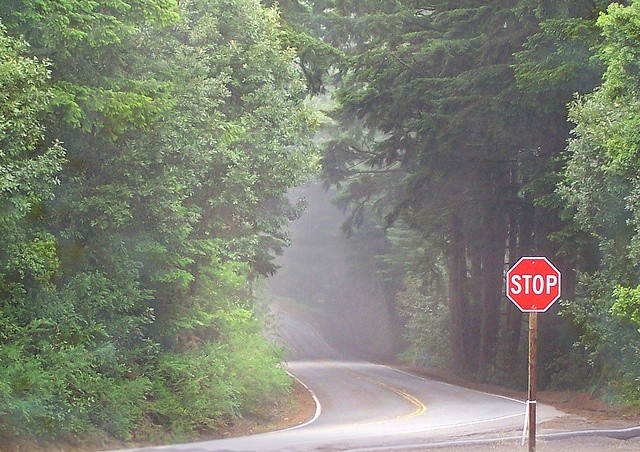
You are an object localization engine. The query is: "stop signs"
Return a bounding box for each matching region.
[507,257,562,312]
